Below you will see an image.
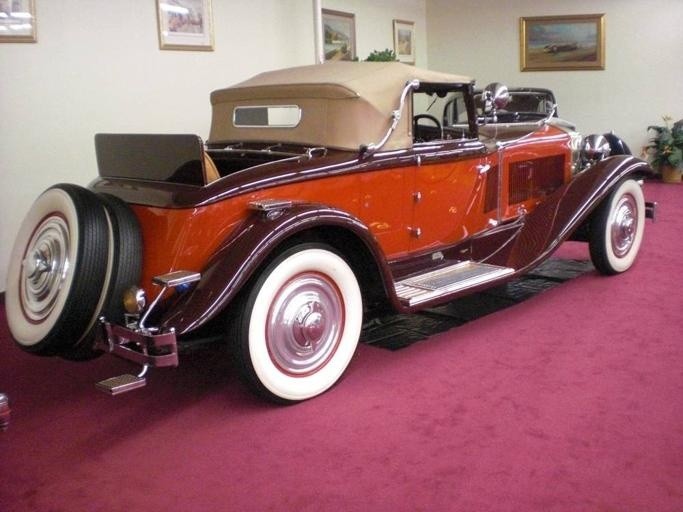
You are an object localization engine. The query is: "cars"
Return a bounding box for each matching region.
[6,61,658,405]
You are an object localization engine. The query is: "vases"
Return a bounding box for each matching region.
[658,163,682,183]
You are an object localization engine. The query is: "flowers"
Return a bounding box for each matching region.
[638,114,683,173]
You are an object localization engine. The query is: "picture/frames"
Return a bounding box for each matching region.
[519,12,607,72]
[321,7,416,66]
[0,0,38,44]
[156,0,216,51]
